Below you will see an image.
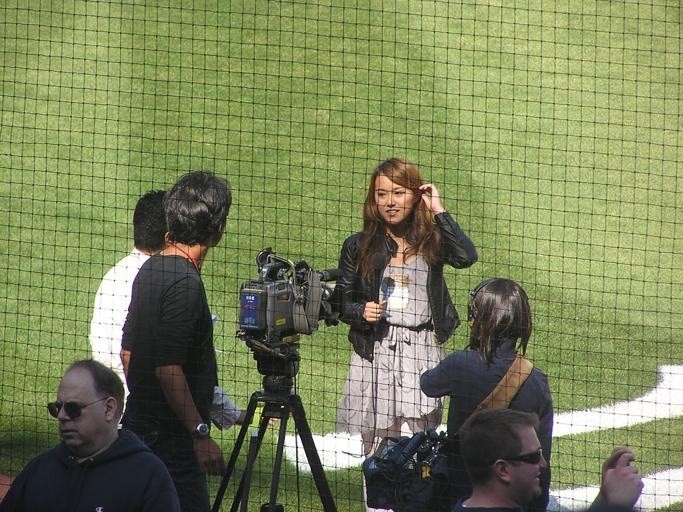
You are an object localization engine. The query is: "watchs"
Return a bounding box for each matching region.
[189,423,211,440]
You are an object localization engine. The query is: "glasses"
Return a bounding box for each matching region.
[506,452,540,465]
[47,395,112,420]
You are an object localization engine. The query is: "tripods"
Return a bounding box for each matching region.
[212,393,338,511]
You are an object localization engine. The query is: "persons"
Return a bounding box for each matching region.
[118,170,232,512]
[417,276,553,511]
[327,157,478,512]
[0,358,182,512]
[87,189,253,432]
[451,408,645,512]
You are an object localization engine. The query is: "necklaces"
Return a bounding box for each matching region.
[170,242,200,272]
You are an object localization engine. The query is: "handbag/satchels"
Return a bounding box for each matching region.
[364,431,449,512]
